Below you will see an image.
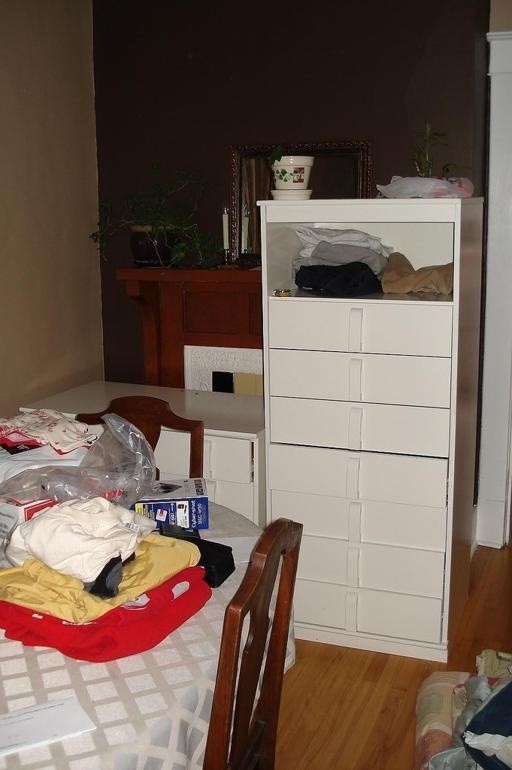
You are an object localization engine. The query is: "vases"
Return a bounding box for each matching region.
[269,155,314,201]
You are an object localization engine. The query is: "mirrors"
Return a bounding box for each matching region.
[230,139,372,272]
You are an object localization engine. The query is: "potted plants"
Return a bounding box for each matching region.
[89,159,223,269]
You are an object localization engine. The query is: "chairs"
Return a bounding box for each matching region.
[203,516,303,769]
[73,394,205,500]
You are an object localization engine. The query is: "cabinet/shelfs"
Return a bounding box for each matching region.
[257,195,486,663]
[117,269,261,388]
[16,380,263,530]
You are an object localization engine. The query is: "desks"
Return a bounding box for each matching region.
[0,467,296,768]
